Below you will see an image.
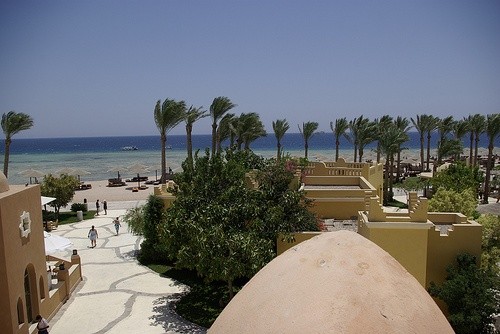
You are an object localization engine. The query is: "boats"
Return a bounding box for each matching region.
[120,144,138,151]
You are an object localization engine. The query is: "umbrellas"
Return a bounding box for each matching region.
[109,163,167,187]
[43,230,74,260]
[18,168,92,184]
[41,197,56,205]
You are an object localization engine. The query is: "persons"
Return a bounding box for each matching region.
[104,201,107,215]
[96,200,100,215]
[114,217,121,235]
[36,315,49,334]
[88,225,98,247]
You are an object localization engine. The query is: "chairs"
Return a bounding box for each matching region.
[45,219,58,230]
[52,260,65,279]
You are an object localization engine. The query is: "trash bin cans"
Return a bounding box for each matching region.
[77,211,82,221]
